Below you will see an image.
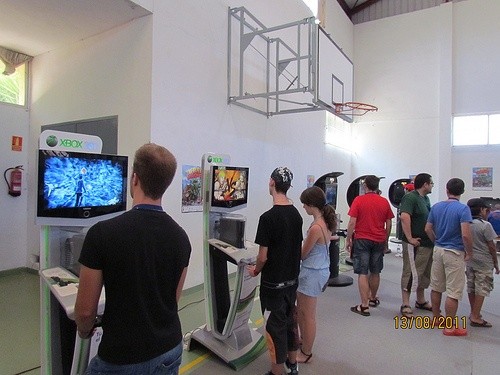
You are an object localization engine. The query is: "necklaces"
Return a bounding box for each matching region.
[449,197,459,201]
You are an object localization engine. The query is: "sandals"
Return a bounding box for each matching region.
[298,349,312,363]
[369,299,379,307]
[400,305,414,319]
[415,301,433,311]
[351,304,370,316]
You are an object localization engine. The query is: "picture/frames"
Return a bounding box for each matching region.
[471,166,493,191]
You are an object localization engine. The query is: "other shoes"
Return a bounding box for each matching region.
[286,359,300,375]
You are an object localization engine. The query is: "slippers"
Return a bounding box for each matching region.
[471,320,491,327]
[443,327,467,336]
[432,317,446,326]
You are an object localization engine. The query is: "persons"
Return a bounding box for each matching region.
[377,189,391,253]
[297,187,338,362]
[245,167,303,375]
[74,141,192,375]
[464,198,500,327]
[487,198,500,252]
[425,178,474,336]
[396,172,442,320]
[346,175,395,316]
[405,184,414,193]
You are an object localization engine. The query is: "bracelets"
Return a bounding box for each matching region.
[254,269,260,276]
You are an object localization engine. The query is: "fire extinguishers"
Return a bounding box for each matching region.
[4,165,24,195]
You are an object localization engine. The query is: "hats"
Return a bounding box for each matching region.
[468,198,489,208]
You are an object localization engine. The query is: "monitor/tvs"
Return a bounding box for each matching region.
[359,180,365,195]
[208,167,250,213]
[325,183,337,210]
[37,149,132,233]
